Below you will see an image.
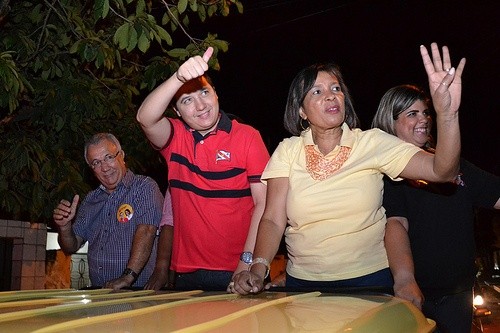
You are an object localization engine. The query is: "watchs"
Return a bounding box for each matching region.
[240,252,253,265]
[124,269,138,279]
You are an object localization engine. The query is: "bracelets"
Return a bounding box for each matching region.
[176,72,186,84]
[248,257,270,278]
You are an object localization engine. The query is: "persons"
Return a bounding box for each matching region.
[372,85,500,333]
[144,188,174,291]
[137,47,269,293]
[234,43,466,296]
[53,134,164,290]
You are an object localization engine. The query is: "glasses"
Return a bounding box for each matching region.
[88,151,119,168]
